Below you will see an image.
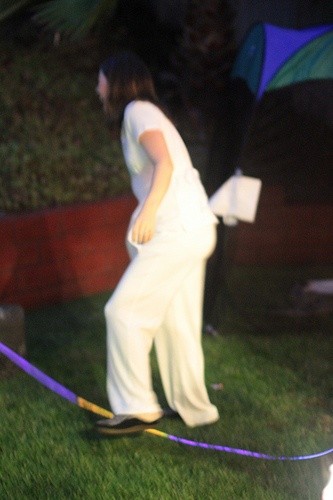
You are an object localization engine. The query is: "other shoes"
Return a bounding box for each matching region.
[166,411,186,424]
[96,413,167,436]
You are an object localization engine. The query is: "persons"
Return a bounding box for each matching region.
[92,56,220,435]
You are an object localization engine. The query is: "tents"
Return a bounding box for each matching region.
[226,16,333,105]
